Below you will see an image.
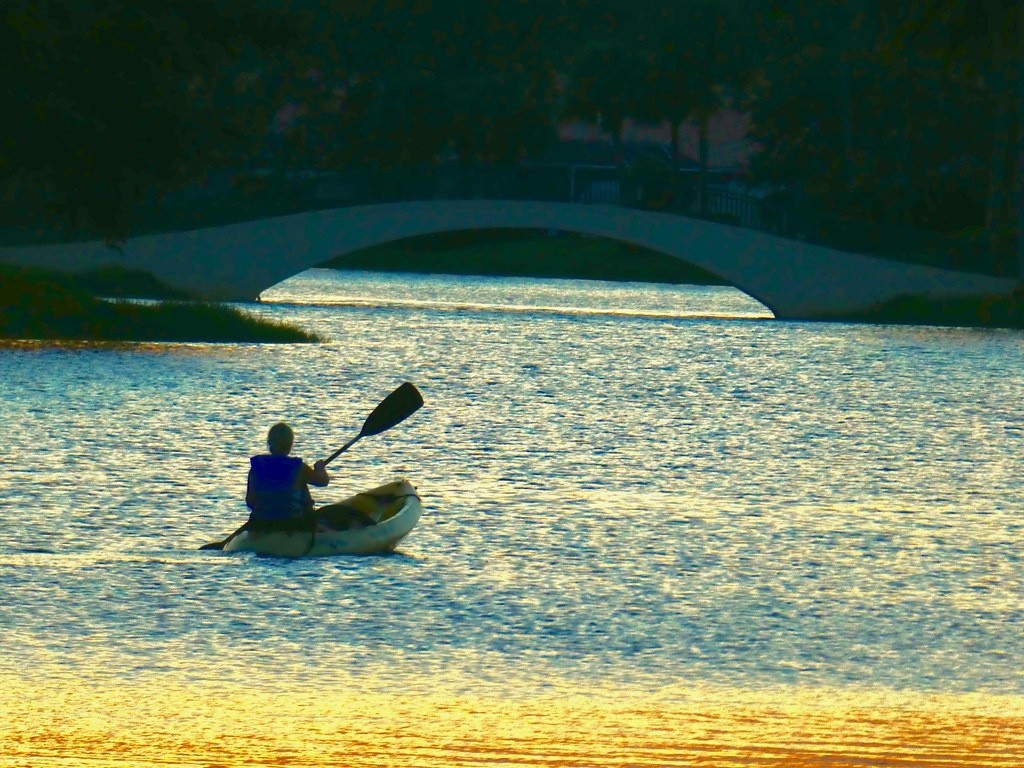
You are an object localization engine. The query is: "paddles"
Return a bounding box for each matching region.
[196,381,424,550]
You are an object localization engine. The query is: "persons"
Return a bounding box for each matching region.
[246,423,330,530]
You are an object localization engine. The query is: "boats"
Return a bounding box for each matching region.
[224,479,422,555]
